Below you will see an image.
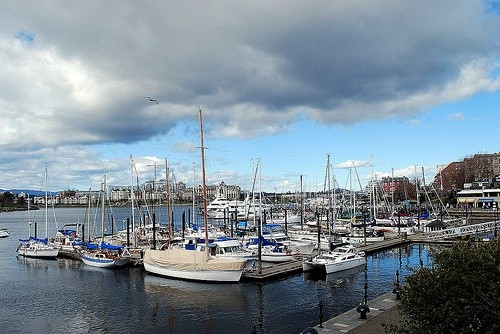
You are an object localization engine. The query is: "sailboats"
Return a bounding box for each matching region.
[13,105,496,282]
